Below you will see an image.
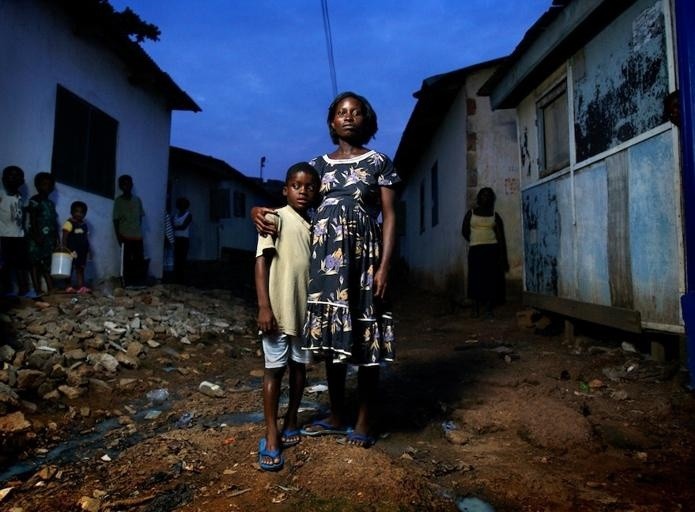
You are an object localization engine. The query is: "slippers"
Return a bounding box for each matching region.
[66,286,92,294]
[259,437,284,471]
[346,432,376,447]
[280,427,300,448]
[299,420,348,436]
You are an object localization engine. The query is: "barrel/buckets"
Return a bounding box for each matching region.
[50,246,74,280]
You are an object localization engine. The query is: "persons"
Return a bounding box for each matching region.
[460,185,511,322]
[253,161,315,472]
[171,193,192,283]
[111,172,150,291]
[0,163,42,308]
[248,90,403,446]
[61,198,95,294]
[25,170,65,297]
